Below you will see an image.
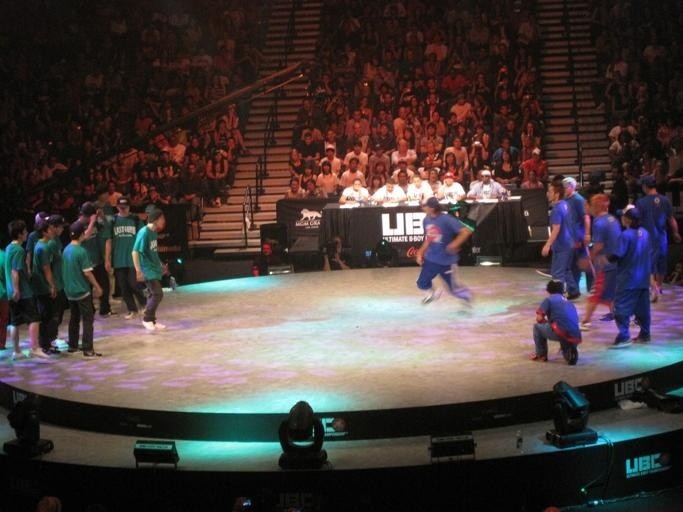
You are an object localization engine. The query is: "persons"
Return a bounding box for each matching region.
[283,1,549,205]
[527,178,682,368]
[588,2,681,211]
[1,196,176,362]
[1,1,256,251]
[413,195,478,305]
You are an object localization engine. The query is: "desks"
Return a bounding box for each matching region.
[333,196,521,269]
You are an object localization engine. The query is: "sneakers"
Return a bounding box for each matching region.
[12,339,102,359]
[526,353,547,362]
[577,312,652,349]
[107,304,165,331]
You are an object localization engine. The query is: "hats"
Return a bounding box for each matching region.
[546,280,563,293]
[637,176,657,186]
[616,203,640,220]
[422,196,439,209]
[146,210,162,222]
[34,198,129,236]
